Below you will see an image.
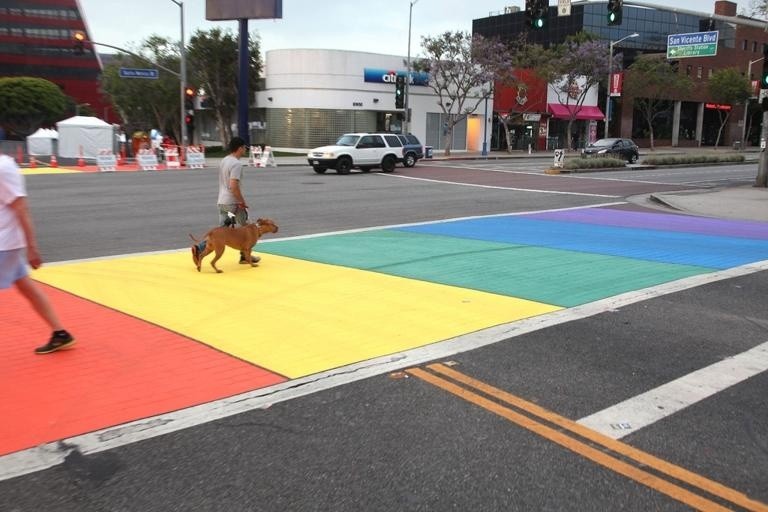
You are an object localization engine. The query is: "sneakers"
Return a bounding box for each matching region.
[240,255,260,264]
[192,245,200,266]
[35,330,75,354]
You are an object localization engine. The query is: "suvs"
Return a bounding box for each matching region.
[397,132,427,168]
[309,134,403,173]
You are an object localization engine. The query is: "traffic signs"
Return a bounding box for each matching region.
[668,31,717,45]
[120,69,159,78]
[666,44,718,57]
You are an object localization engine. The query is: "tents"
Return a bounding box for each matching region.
[55,115,116,165]
[26,127,59,166]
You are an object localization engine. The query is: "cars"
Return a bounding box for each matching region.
[582,137,639,163]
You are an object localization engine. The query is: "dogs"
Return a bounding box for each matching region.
[190,218,279,273]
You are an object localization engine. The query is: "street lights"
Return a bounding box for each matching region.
[482,92,490,156]
[74,33,188,164]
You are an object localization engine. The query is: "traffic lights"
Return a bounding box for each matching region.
[535,0,548,28]
[185,117,193,125]
[604,32,641,136]
[607,1,624,25]
[395,77,404,108]
[185,86,195,109]
[525,0,534,26]
[763,45,768,89]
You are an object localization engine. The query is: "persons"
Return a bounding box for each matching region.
[0,147,74,356]
[214,136,260,265]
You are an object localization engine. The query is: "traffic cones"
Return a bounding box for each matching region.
[48,151,59,166]
